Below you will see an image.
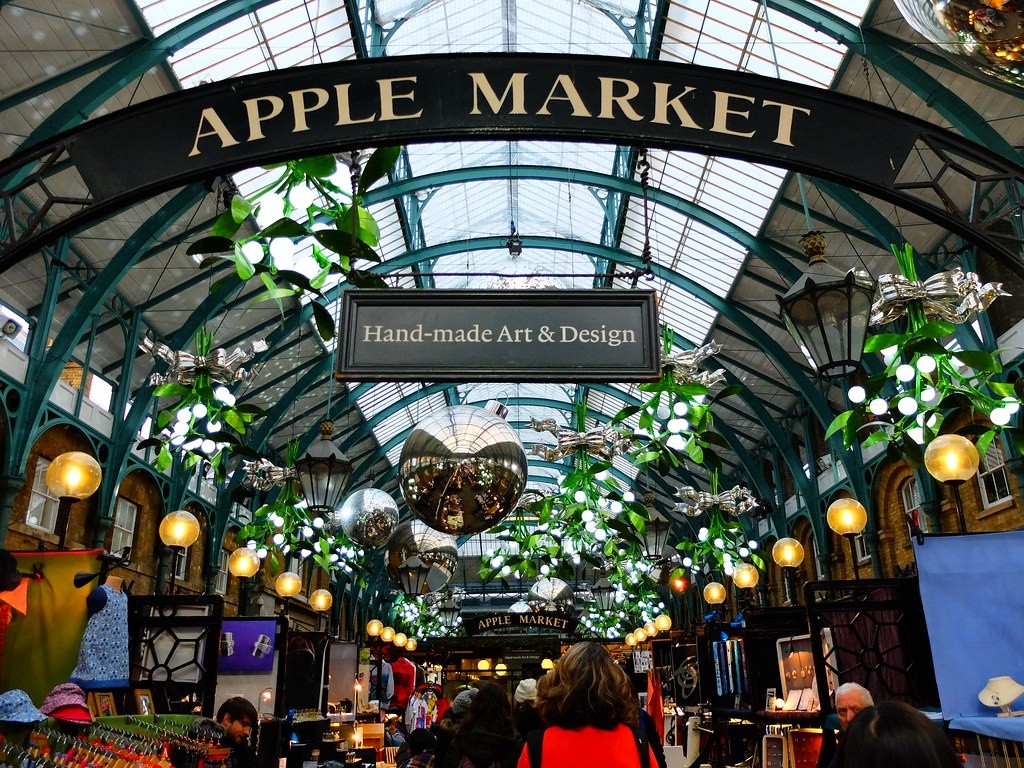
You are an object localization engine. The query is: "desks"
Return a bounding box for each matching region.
[327,711,378,739]
[259,718,331,768]
[751,709,819,768]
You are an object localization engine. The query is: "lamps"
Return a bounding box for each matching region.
[43,0,985,678]
[73,554,131,588]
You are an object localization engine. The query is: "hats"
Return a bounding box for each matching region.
[39,683,92,725]
[0,689,48,722]
[515,678,538,702]
[452,689,479,713]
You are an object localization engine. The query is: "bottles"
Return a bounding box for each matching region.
[296,708,322,720]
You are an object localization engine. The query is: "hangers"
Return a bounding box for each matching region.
[398,652,435,699]
[0,716,228,768]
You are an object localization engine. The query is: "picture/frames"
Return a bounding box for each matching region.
[93,692,117,717]
[133,688,156,715]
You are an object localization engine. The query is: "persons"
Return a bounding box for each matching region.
[517,643,659,768]
[831,701,964,768]
[386,678,667,768]
[815,682,875,768]
[217,696,258,744]
